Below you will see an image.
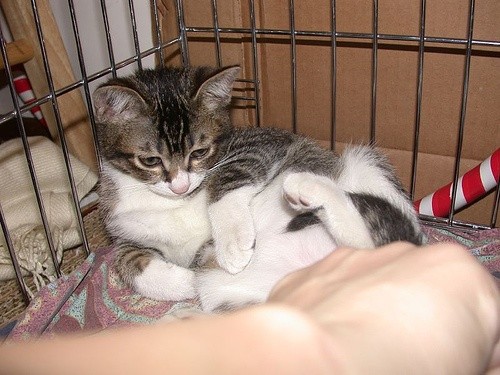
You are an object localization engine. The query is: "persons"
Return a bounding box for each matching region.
[0,241,500,375]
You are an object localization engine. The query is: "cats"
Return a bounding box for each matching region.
[93,63,427,316]
